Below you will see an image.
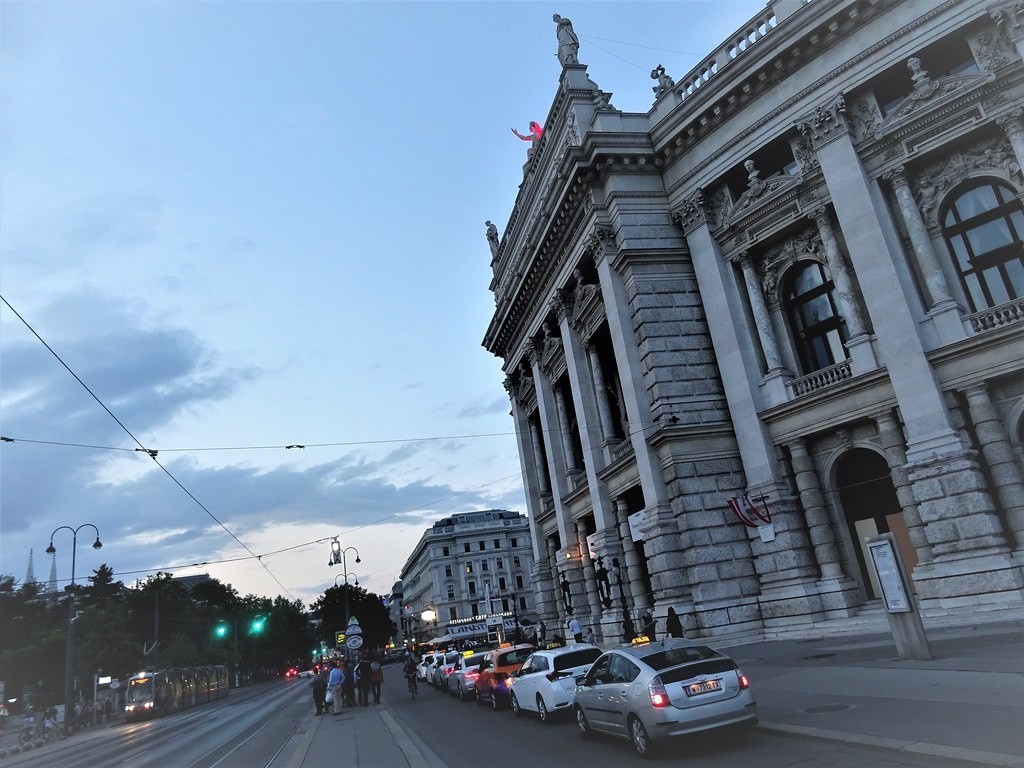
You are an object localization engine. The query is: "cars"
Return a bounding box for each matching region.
[508,586,522,646]
[509,643,604,724]
[474,642,537,710]
[425,654,445,684]
[445,651,488,703]
[377,654,403,666]
[415,654,434,682]
[285,659,337,679]
[433,650,463,691]
[572,638,758,759]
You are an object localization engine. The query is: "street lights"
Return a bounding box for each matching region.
[46,523,103,737]
[611,556,639,643]
[328,546,361,662]
[218,615,262,687]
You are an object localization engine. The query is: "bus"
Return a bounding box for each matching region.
[125,665,229,722]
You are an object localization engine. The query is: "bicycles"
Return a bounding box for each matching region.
[17,720,67,744]
[403,671,420,700]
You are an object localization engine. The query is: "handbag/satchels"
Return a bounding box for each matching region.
[325,690,333,703]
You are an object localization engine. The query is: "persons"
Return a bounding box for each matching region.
[309,617,595,715]
[552,14,580,67]
[666,606,684,639]
[804,240,827,264]
[94,697,112,724]
[541,323,552,337]
[743,160,760,180]
[762,257,781,300]
[518,363,527,375]
[906,57,928,80]
[650,64,674,100]
[511,121,543,148]
[975,149,1022,182]
[573,268,585,284]
[641,609,656,641]
[914,176,948,230]
[485,220,499,258]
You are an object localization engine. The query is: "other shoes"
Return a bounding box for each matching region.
[332,712,341,716]
[313,712,321,716]
